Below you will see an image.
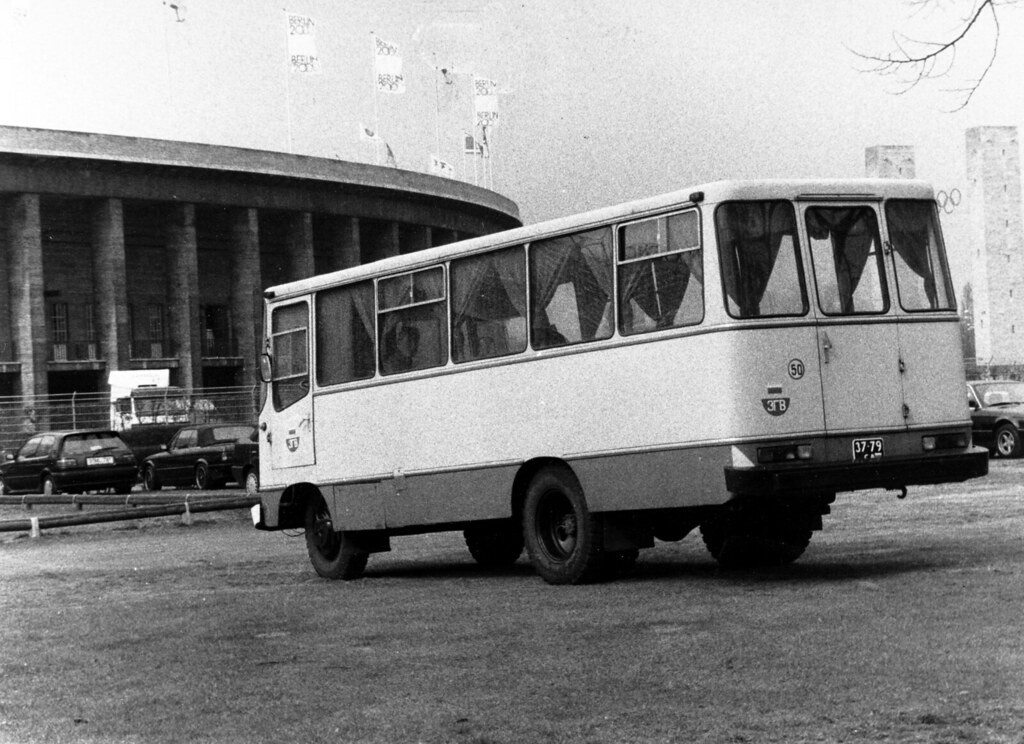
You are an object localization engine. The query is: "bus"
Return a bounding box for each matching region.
[250,177,992,587]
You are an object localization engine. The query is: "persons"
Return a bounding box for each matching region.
[382,325,422,374]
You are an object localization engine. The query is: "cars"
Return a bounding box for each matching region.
[966,379,1024,459]
[0,427,141,496]
[142,423,260,493]
